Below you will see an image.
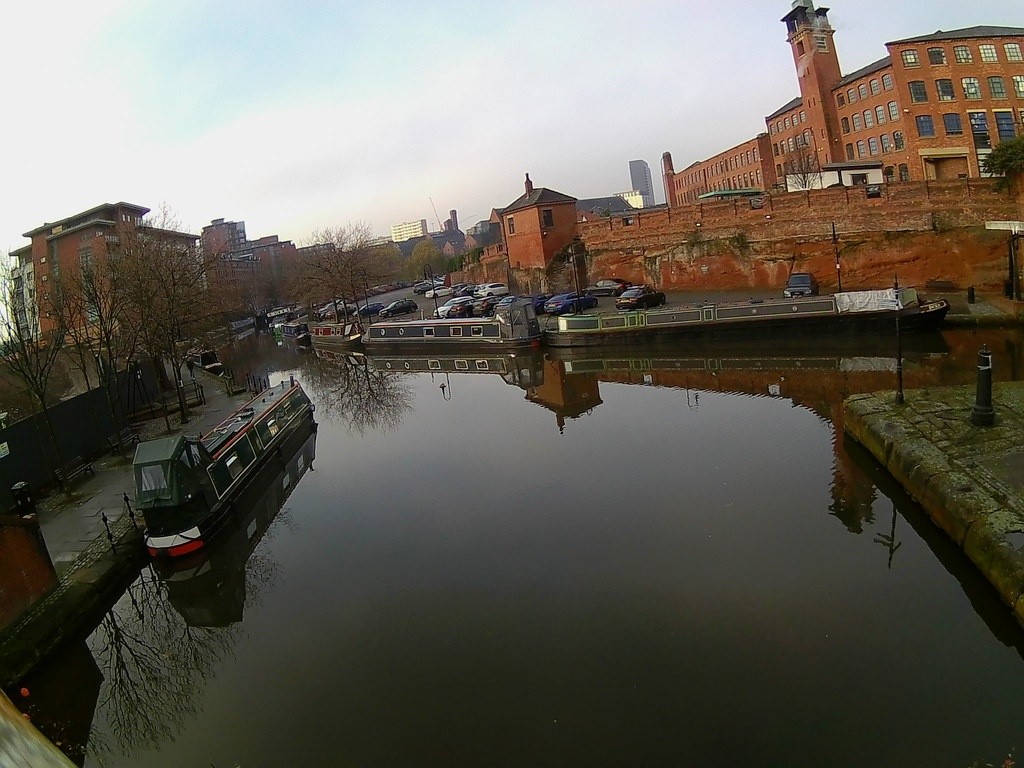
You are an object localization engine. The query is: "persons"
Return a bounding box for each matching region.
[187,357,195,380]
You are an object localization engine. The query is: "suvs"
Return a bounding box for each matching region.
[783,272,818,297]
[413,277,557,319]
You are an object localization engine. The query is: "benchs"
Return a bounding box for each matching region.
[53,455,95,492]
[108,426,143,456]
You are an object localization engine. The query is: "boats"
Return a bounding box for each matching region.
[187,348,223,372]
[311,341,368,365]
[543,329,951,375]
[542,289,951,348]
[131,372,317,549]
[311,319,364,348]
[148,414,322,629]
[361,293,543,355]
[366,347,546,391]
[283,324,311,342]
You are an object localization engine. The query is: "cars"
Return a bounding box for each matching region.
[581,278,632,297]
[615,285,666,311]
[313,301,357,320]
[543,292,599,314]
[378,297,418,319]
[352,301,384,319]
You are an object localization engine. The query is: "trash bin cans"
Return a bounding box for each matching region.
[1002,279,1009,297]
[11,481,36,516]
